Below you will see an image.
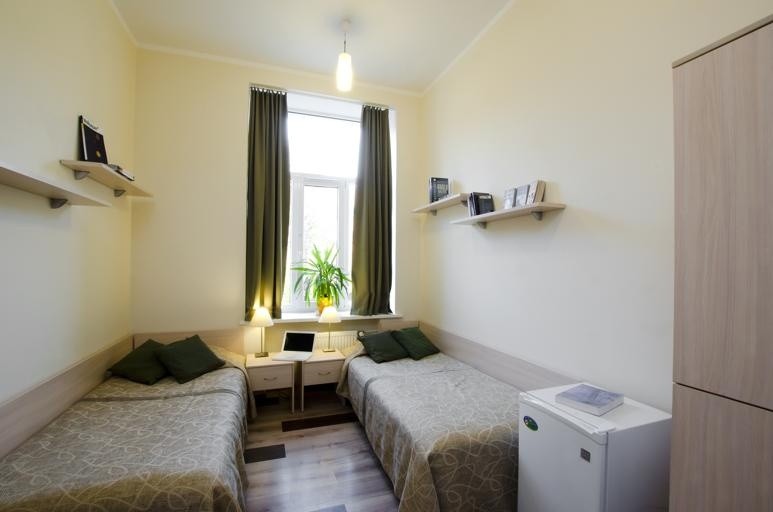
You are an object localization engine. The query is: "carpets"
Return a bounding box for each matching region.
[242,394,400,512]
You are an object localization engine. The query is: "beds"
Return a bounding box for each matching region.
[0,327,265,512]
[337,344,527,512]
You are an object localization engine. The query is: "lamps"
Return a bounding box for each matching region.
[336,20,353,90]
[318,307,342,352]
[249,308,273,358]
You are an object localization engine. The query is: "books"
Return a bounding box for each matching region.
[430,177,449,203]
[106,163,135,181]
[515,184,530,207]
[80,115,108,164]
[526,180,545,204]
[469,192,494,216]
[501,188,517,209]
[556,384,625,417]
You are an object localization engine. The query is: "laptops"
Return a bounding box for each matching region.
[273,332,315,361]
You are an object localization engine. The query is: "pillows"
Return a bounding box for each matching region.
[358,331,408,364]
[390,327,440,360]
[154,334,226,385]
[106,339,171,385]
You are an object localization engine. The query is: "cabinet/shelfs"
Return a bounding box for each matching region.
[670,16,773,512]
[518,383,672,512]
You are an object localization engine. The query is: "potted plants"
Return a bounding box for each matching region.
[289,239,352,315]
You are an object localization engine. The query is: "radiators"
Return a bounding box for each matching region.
[315,331,358,351]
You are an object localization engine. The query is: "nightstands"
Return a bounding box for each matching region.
[302,348,346,412]
[245,353,295,414]
